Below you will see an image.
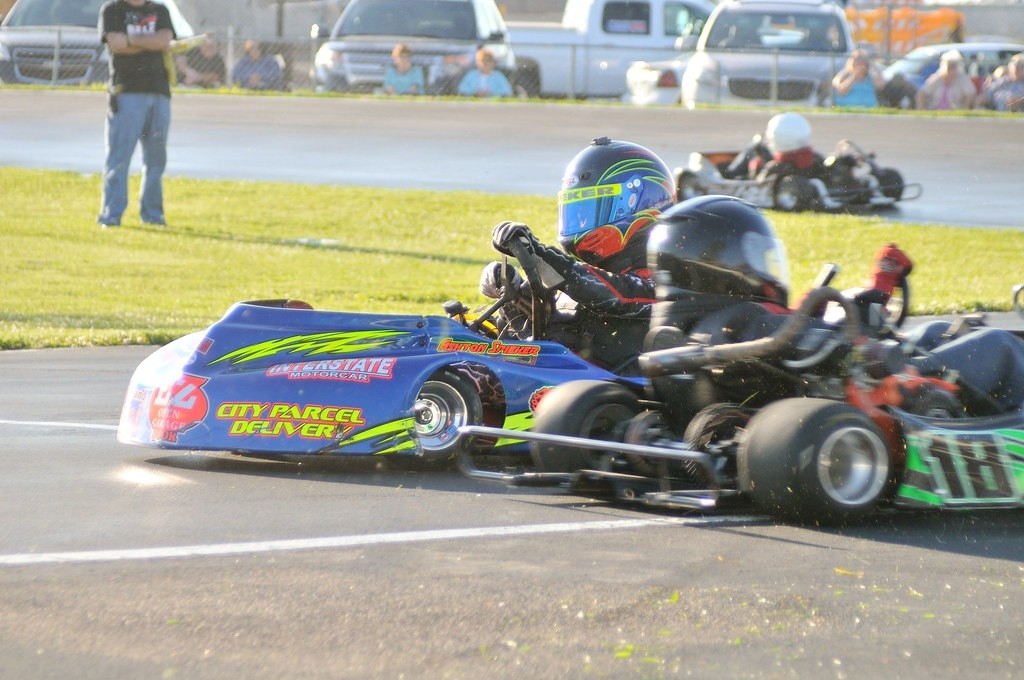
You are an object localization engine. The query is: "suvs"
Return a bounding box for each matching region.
[0,0,193,86]
[620,0,878,107]
[311,0,519,92]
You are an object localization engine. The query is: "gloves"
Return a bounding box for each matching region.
[871,244,911,298]
[494,223,548,256]
[481,262,525,301]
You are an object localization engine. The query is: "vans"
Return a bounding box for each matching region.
[881,43,1024,91]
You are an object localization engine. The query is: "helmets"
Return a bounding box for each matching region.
[766,113,813,159]
[558,136,677,247]
[647,196,789,309]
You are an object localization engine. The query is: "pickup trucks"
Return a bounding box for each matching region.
[505,0,716,96]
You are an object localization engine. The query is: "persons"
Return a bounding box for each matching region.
[385,43,424,95]
[917,50,977,110]
[984,52,1024,111]
[647,194,913,337]
[97,0,177,227]
[720,112,812,179]
[480,137,678,370]
[176,38,224,88]
[235,38,284,91]
[833,48,878,107]
[459,49,512,98]
